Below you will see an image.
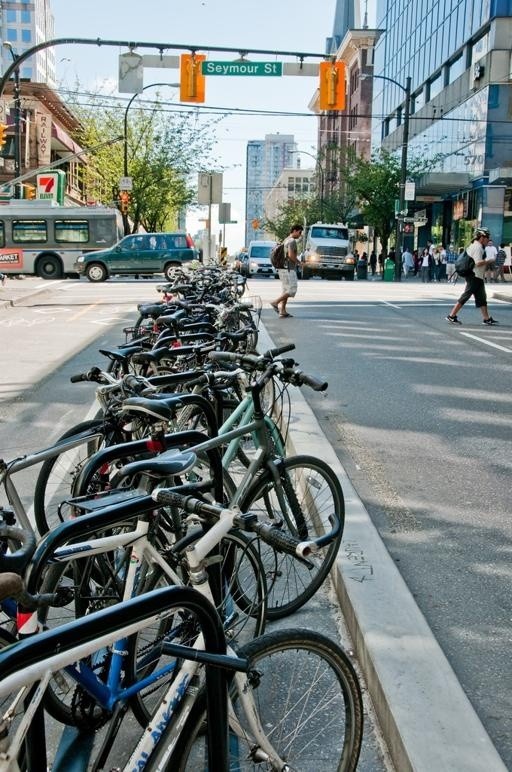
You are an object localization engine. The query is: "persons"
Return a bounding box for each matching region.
[446,226,500,327]
[269,224,306,321]
[349,237,512,287]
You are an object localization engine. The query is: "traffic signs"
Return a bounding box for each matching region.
[200,59,283,78]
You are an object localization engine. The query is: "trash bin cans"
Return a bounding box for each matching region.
[357,259,367,279]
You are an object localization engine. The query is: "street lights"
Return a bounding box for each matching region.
[118,80,183,262]
[352,72,413,282]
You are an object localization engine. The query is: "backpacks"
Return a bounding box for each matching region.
[271,238,297,268]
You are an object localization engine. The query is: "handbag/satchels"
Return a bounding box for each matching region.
[455,250,475,277]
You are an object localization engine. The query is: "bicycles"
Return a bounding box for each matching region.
[0,261,365,772]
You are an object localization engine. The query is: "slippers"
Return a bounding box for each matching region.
[279,313,293,317]
[271,303,279,313]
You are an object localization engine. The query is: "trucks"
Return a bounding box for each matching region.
[298,222,359,284]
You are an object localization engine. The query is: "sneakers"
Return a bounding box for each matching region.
[446,314,461,324]
[482,316,498,325]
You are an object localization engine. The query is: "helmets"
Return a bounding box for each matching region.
[476,228,491,236]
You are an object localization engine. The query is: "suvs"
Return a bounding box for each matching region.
[76,223,202,288]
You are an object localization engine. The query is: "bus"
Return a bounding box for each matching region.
[2,200,120,280]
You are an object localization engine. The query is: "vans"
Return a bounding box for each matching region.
[249,241,281,280]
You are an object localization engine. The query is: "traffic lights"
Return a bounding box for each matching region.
[0,124,11,149]
